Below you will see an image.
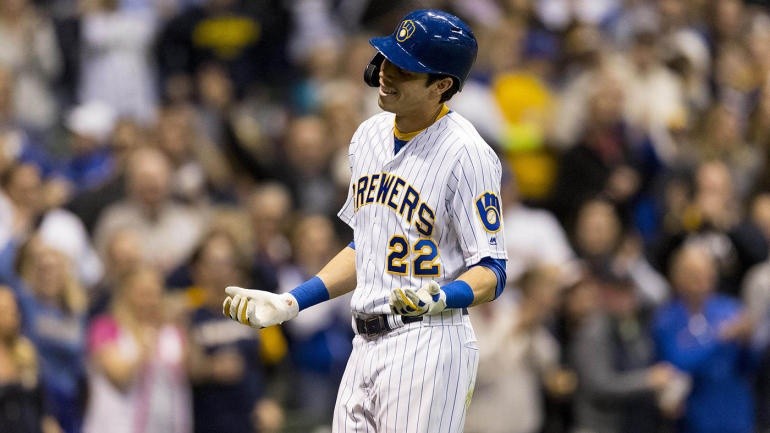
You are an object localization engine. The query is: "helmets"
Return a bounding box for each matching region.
[362,9,477,106]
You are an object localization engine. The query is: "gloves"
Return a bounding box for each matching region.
[220,283,299,327]
[388,280,447,319]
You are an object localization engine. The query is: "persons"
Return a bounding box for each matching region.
[221,9,510,433]
[0,0,770,433]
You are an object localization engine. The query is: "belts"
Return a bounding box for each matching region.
[355,310,422,339]
